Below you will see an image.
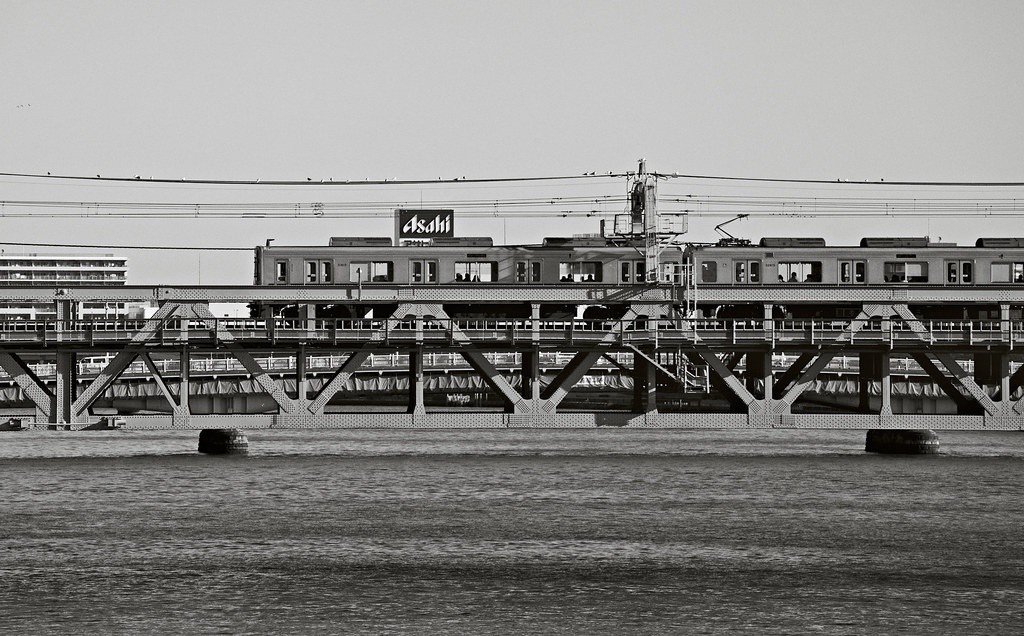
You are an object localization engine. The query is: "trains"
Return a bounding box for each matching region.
[246,212,1024,334]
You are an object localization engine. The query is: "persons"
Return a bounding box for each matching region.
[1017,275,1024,283]
[560,274,597,281]
[841,263,864,282]
[885,275,905,282]
[456,273,480,282]
[778,272,821,282]
